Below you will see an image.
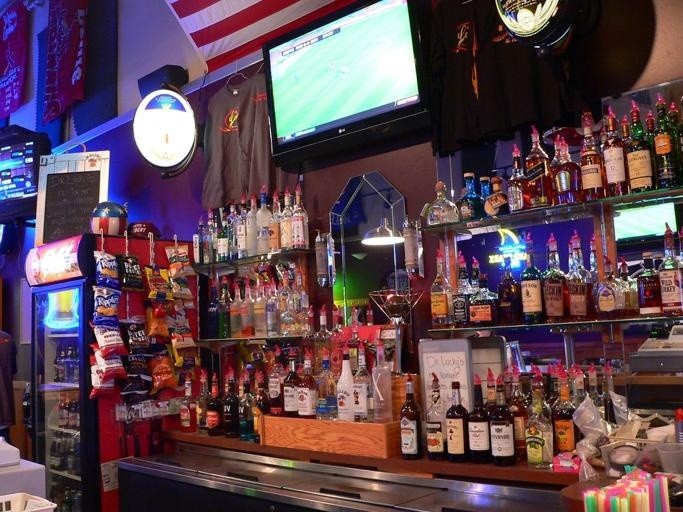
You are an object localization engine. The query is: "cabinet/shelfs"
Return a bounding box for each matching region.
[400,177,683,494]
[43,332,85,510]
[185,240,333,442]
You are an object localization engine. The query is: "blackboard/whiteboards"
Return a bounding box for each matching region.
[33,150,110,248]
[418,338,474,422]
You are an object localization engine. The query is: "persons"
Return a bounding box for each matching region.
[0,329,17,445]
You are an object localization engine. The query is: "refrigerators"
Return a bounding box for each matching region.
[23,234,198,512]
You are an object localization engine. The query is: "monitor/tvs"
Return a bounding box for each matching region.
[261,1,433,163]
[0,124,51,226]
[614,202,681,248]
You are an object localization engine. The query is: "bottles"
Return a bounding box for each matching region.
[179,91,683,468]
[50,338,85,509]
[53,290,76,322]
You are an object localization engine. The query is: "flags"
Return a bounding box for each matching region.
[0,1,27,120]
[164,0,360,76]
[42,1,87,126]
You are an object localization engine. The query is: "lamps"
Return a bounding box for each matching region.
[358,214,410,297]
